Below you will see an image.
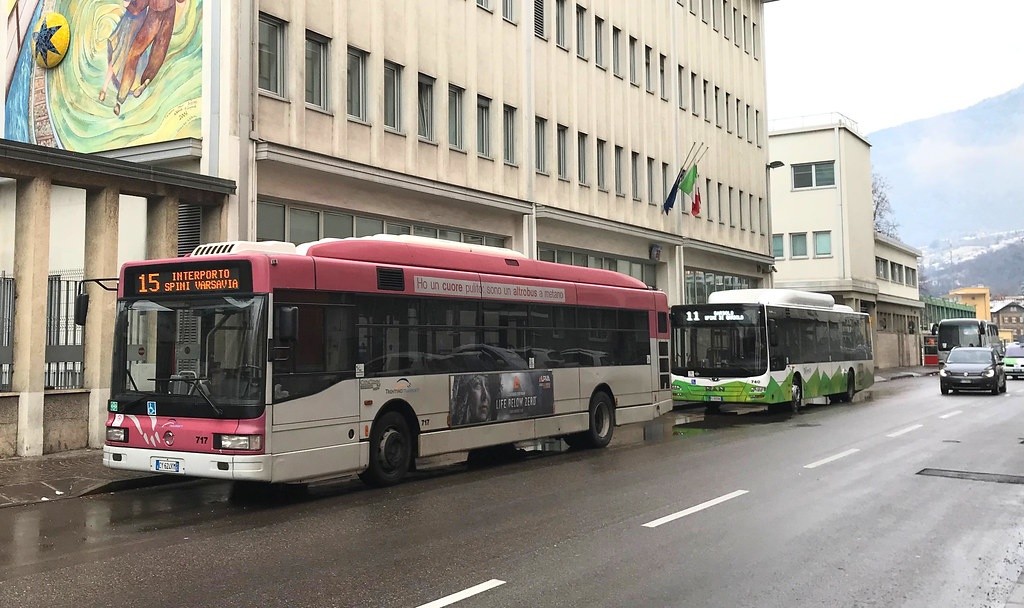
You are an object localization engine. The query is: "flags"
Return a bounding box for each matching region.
[677,162,702,216]
[663,166,684,216]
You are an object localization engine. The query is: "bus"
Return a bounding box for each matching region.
[670,287,876,415]
[931,318,999,372]
[71,233,675,495]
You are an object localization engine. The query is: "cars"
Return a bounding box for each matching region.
[1002,343,1024,379]
[938,345,1007,395]
[361,341,613,378]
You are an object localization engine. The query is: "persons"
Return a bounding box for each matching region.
[449,374,503,424]
